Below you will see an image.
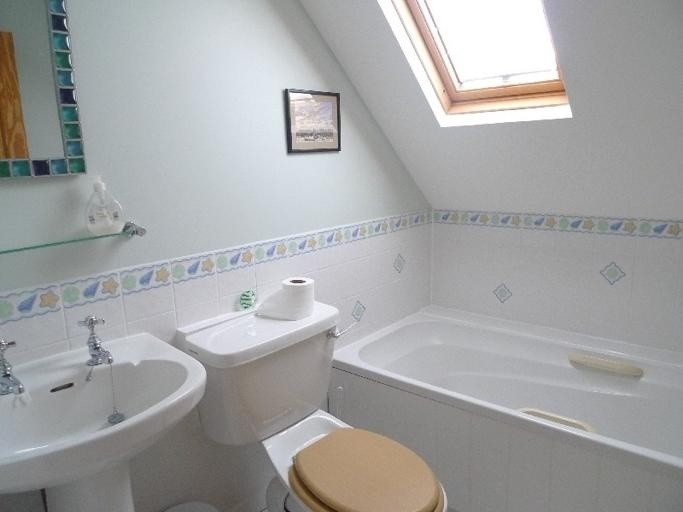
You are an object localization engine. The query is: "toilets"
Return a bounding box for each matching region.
[174,298,449,511]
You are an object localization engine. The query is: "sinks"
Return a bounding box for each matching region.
[0,331,208,492]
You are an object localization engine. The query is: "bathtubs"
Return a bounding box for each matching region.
[330,304,683,483]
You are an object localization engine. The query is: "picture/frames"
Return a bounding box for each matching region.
[285,88,341,153]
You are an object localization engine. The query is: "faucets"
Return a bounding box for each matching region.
[75,314,115,368]
[1,336,24,396]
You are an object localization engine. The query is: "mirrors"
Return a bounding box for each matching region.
[0,1,86,178]
